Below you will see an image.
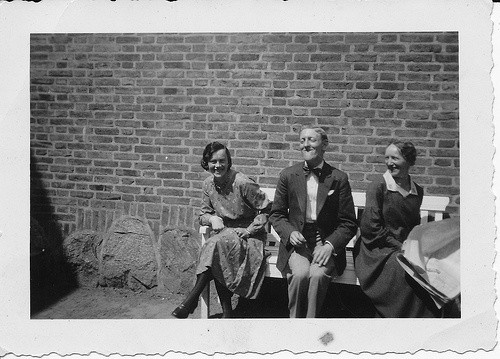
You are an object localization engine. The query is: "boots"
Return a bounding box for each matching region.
[214,279,234,319]
[170,264,213,319]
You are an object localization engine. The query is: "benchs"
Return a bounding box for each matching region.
[199,188,450,318]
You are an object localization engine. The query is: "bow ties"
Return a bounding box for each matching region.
[302,166,322,178]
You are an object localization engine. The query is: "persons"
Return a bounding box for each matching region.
[171,142,273,318]
[271,125,360,318]
[353,139,461,318]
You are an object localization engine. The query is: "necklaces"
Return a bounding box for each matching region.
[214,176,229,191]
